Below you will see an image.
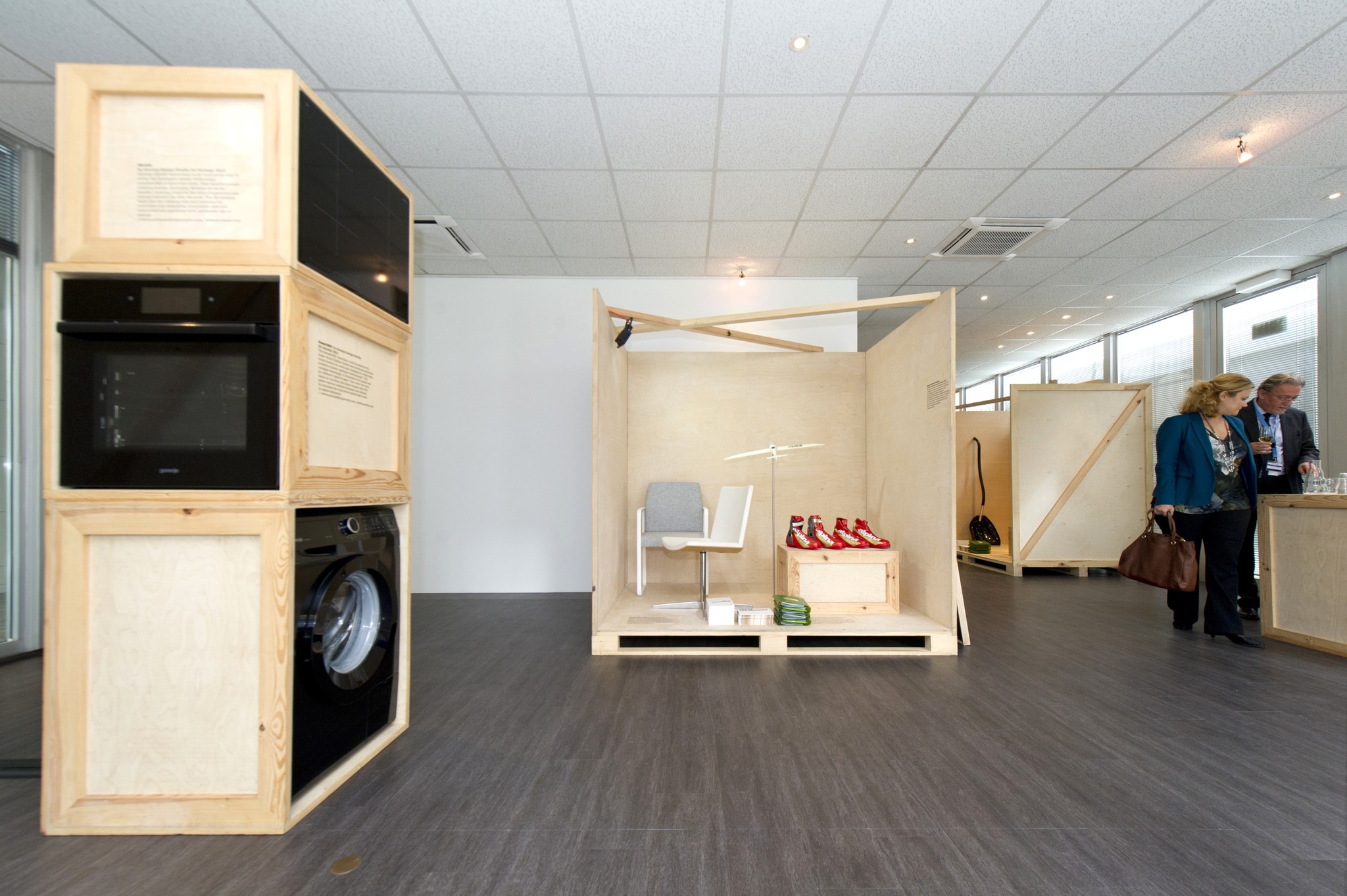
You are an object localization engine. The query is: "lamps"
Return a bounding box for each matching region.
[1237,135,1254,165]
[738,265,748,279]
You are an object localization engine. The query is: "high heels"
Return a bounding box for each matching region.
[1212,628,1264,648]
[1172,619,1193,630]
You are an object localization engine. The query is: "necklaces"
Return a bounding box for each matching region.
[1201,409,1235,472]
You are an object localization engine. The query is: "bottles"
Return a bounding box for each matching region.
[1303,459,1329,494]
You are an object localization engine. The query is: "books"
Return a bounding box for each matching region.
[705,596,776,628]
[957,540,970,552]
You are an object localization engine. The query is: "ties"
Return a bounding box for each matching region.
[1261,414,1271,445]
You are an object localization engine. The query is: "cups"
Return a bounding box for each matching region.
[1325,472,1347,494]
[1258,427,1273,452]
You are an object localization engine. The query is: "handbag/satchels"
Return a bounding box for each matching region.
[1118,508,1197,595]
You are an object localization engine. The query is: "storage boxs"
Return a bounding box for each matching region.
[36,497,414,838]
[49,60,416,335]
[40,260,417,504]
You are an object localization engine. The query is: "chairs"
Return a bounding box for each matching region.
[650,483,754,621]
[635,481,710,597]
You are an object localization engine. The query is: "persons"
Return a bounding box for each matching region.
[1147,372,1267,649]
[1236,372,1321,625]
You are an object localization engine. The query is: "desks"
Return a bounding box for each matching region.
[1256,491,1346,658]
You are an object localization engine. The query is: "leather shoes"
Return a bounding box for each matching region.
[1238,607,1259,621]
